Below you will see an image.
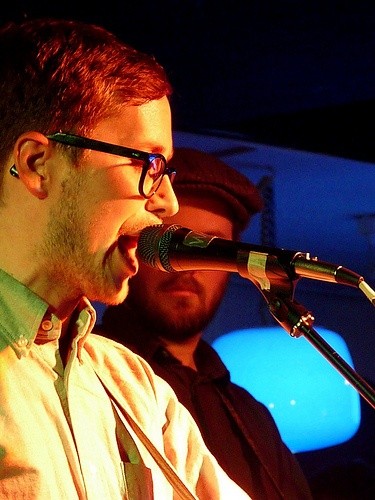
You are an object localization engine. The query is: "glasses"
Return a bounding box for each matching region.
[9,132,177,200]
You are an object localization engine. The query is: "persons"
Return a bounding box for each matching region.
[89,149,312,500]
[0,20,249,500]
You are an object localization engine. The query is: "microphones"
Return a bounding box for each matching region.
[136,224,364,288]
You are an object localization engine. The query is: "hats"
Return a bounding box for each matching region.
[165,147,264,234]
